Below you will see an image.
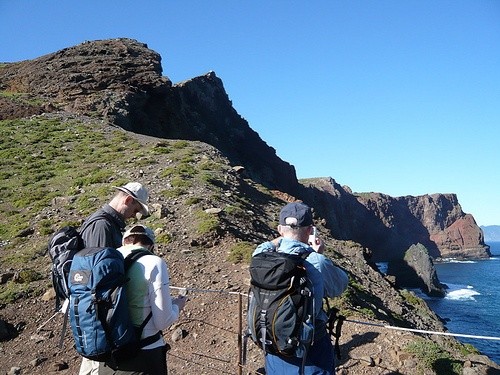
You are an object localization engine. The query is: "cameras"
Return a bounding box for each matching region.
[308,226,317,243]
[177,289,188,297]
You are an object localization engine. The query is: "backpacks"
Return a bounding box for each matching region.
[246,242,311,358]
[47,216,110,312]
[62,246,156,362]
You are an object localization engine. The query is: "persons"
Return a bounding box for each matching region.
[251,200,349,375]
[76,181,152,375]
[111,223,191,375]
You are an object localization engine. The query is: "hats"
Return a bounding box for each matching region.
[279,202,313,228]
[121,224,154,250]
[112,182,151,217]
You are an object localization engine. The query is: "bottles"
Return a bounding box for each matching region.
[295,315,315,358]
